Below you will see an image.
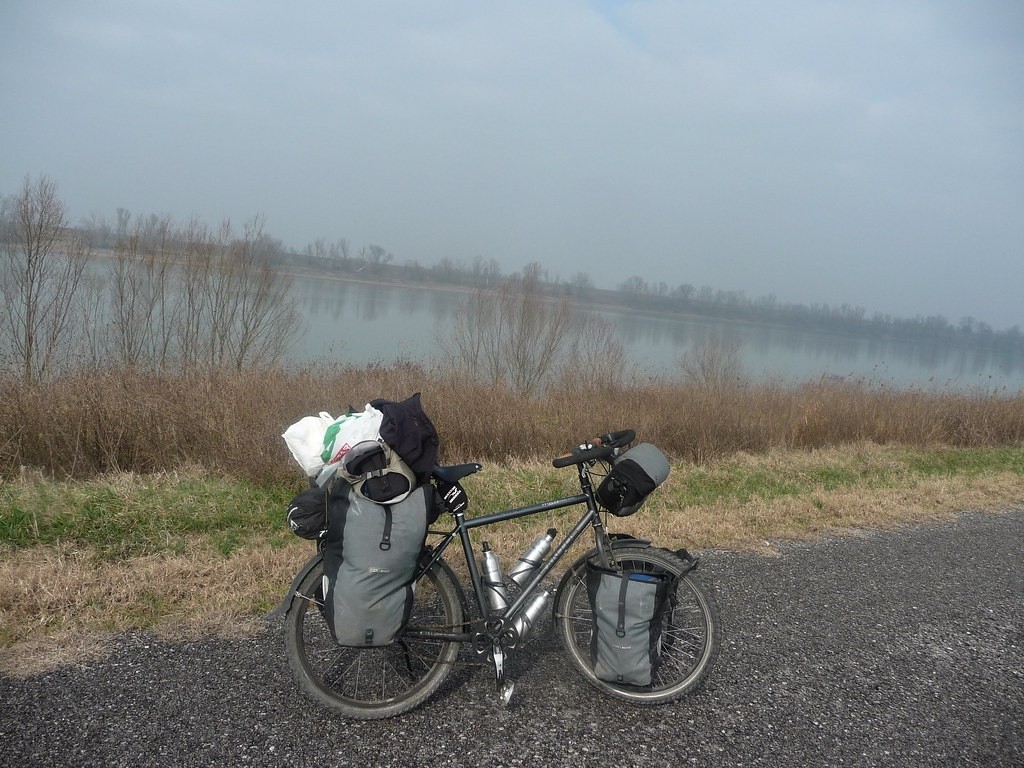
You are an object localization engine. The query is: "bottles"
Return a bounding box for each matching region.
[482,540,509,611]
[507,528,558,588]
[506,585,556,649]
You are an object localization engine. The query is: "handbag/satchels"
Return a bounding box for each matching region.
[340,442,415,504]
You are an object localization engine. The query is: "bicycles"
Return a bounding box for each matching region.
[263,423,723,720]
